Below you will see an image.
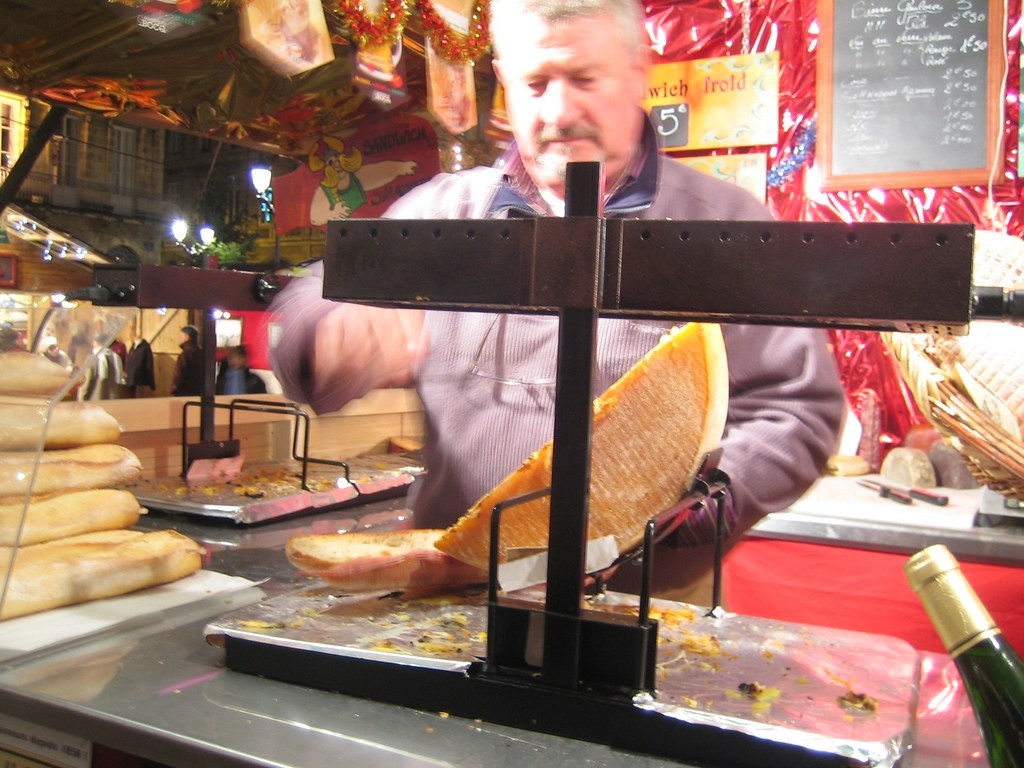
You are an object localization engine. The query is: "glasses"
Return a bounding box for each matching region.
[469,312,558,386]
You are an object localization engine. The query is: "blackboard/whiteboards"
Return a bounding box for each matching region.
[814,0,1007,195]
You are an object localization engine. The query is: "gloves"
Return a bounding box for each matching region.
[584,481,746,595]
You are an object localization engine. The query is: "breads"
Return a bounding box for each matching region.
[284,528,495,589]
[432,320,728,576]
[0,349,203,621]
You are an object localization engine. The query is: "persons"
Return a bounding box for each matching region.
[171,326,204,396]
[268,0,847,611]
[0,321,127,401]
[216,345,266,394]
[126,334,156,390]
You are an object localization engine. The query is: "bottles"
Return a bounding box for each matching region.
[907,544,1024,768]
